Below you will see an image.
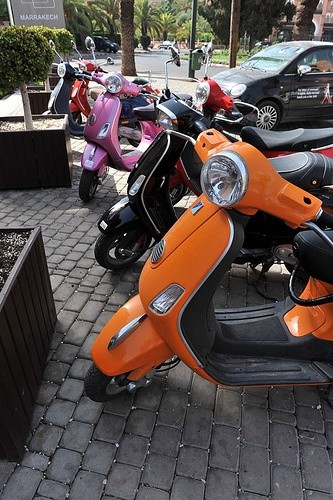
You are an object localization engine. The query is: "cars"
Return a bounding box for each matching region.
[158,41,178,50]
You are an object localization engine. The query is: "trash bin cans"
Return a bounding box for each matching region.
[191,49,203,71]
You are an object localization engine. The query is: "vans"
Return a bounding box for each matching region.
[210,41,333,131]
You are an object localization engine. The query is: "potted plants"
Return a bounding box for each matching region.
[49,28,75,86]
[0,24,73,190]
[27,26,60,115]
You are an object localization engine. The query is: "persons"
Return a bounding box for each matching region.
[200,43,208,64]
[163,28,169,42]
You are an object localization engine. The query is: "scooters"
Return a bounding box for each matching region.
[94,46,333,301]
[84,120,333,402]
[41,36,194,202]
[166,41,333,206]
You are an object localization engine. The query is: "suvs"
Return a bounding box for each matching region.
[93,36,119,53]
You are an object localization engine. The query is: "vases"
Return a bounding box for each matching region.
[0,225,57,462]
